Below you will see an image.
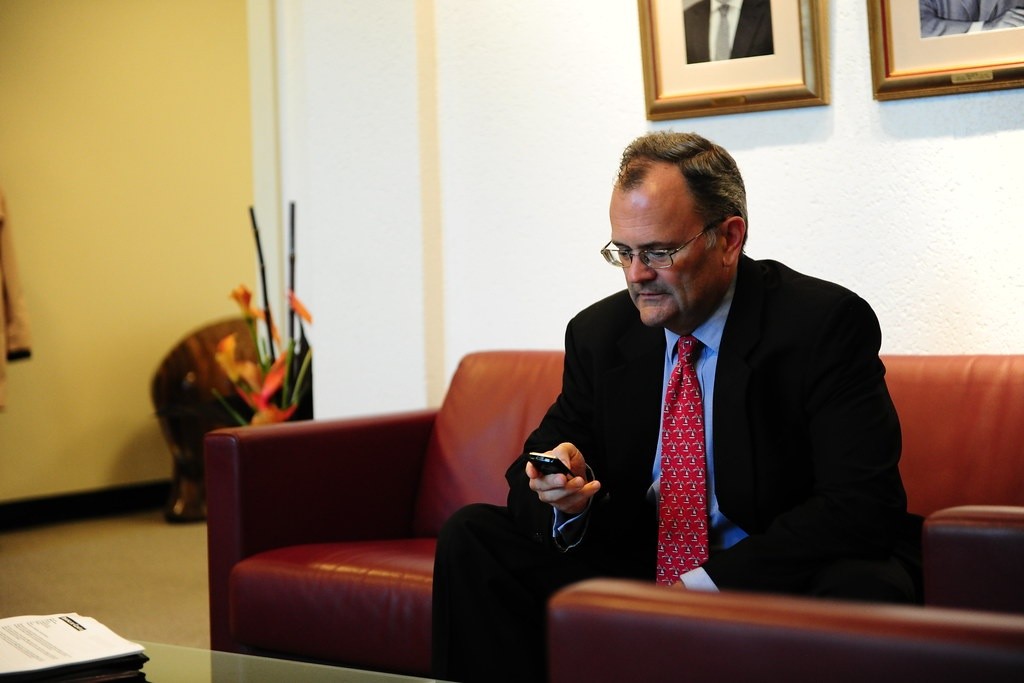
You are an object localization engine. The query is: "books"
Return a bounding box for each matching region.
[0,612,149,683]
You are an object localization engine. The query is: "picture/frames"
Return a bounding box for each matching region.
[636,0,829,123]
[865,0,1024,114]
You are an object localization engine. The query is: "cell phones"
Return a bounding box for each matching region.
[529,452,576,481]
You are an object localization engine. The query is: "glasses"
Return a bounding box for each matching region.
[601,215,735,269]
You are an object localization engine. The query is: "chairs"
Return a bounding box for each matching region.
[549,504,1024,683]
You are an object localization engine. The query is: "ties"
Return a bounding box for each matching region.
[655,334,709,589]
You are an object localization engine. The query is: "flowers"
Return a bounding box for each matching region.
[214,287,312,427]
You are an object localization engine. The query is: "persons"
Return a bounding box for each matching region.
[431,132,915,683]
[919,0,1024,38]
[683,0,774,64]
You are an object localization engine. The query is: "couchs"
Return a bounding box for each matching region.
[207,350,1024,683]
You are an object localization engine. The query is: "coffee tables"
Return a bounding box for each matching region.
[1,641,455,683]
